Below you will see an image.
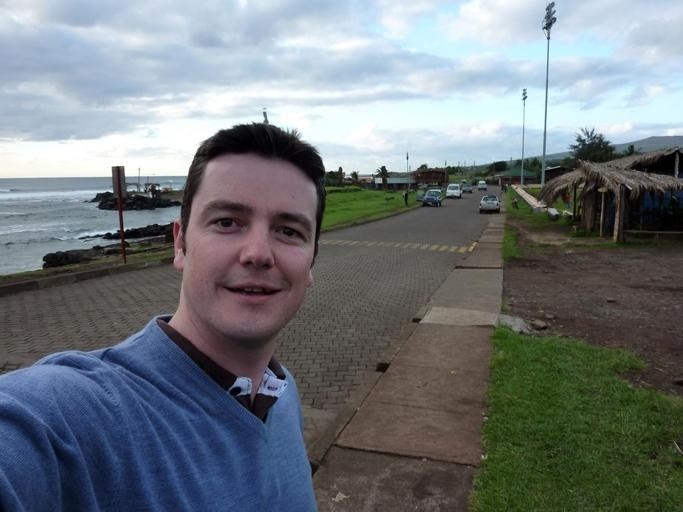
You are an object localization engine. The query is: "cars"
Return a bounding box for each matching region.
[479,194,501,213]
[461,183,472,193]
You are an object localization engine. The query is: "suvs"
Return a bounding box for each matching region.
[422,188,443,207]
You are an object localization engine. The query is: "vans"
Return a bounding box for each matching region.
[446,184,463,198]
[477,181,488,190]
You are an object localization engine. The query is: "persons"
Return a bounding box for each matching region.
[405,190,408,206]
[0,122,326,512]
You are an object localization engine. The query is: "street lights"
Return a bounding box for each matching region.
[540,1,558,187]
[520,89,529,185]
